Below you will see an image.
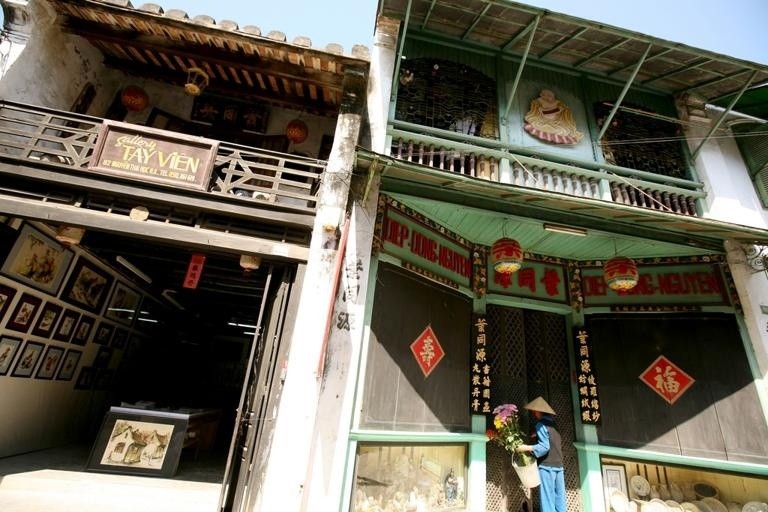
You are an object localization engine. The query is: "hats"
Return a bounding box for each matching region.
[521,395,558,417]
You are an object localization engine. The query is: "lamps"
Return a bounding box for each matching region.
[183,64,210,97]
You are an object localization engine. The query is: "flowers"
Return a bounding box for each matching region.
[486,402,536,466]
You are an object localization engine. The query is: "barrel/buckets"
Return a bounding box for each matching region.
[511,448,541,488]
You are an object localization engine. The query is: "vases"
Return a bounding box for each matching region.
[511,460,543,490]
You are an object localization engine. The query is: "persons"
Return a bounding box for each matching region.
[515,410,567,512]
[526,90,584,145]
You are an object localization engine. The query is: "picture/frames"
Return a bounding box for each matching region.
[83,408,189,479]
[1,219,167,392]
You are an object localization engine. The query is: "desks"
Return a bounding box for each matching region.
[109,405,225,465]
